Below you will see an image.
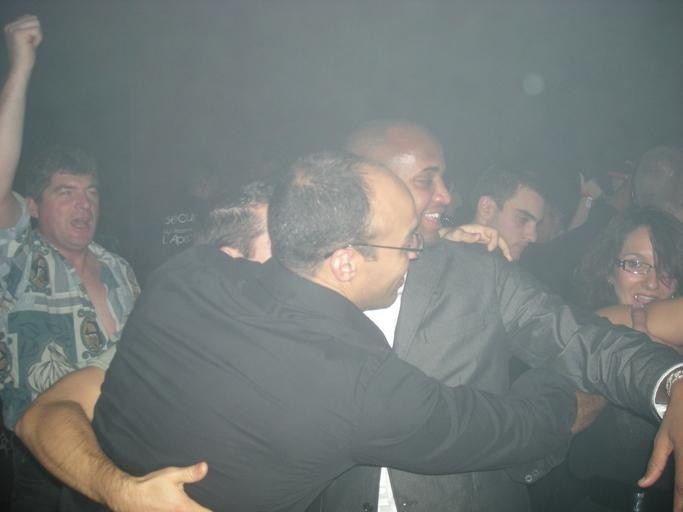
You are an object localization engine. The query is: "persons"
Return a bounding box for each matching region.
[343,117,683,512]
[0,12,44,248]
[566,205,683,512]
[1,144,142,512]
[467,143,683,308]
[59,151,578,512]
[14,170,513,512]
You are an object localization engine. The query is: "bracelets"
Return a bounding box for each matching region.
[666,370,683,398]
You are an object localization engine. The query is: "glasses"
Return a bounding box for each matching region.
[615,255,655,275]
[323,234,425,260]
